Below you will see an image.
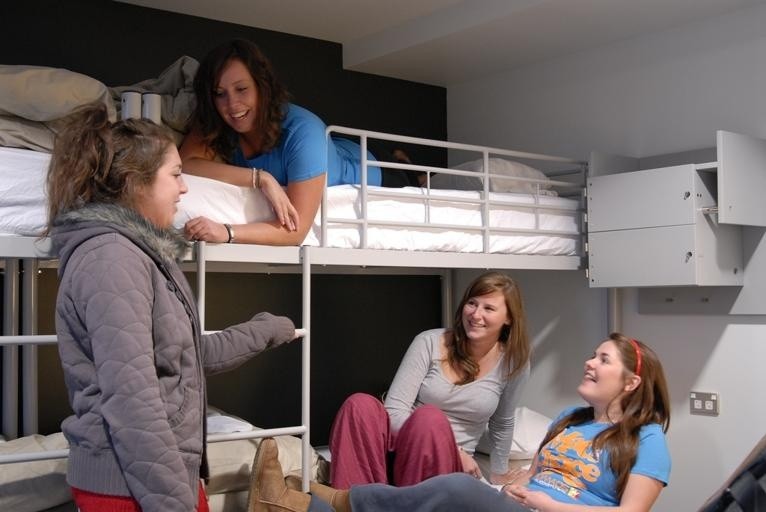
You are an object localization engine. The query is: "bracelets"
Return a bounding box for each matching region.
[223,223,234,244]
[257,168,263,189]
[251,167,257,190]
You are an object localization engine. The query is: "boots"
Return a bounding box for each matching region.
[247,437,311,511]
[285,475,350,512]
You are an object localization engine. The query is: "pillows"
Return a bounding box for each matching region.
[420,157,564,199]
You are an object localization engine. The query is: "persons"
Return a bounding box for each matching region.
[328,269,536,490]
[31,102,296,512]
[177,37,435,246]
[242,332,672,512]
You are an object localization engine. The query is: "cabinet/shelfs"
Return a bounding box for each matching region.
[588,130,766,289]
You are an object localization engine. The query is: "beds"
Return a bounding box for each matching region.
[1,403,579,510]
[0,123,583,281]
